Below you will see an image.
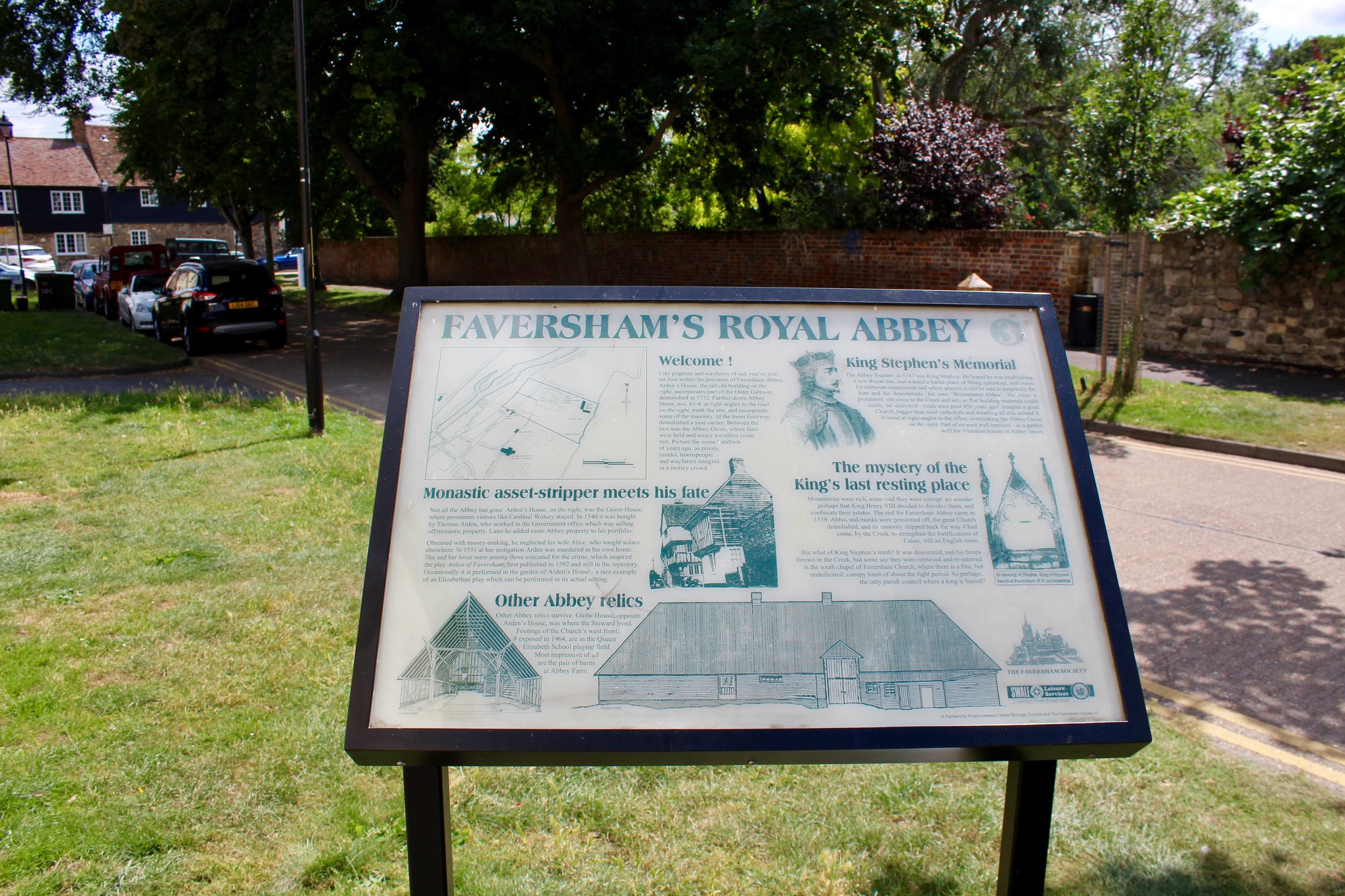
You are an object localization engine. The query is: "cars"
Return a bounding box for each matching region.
[66,258,109,312]
[0,261,38,311]
[255,246,304,273]
[151,255,289,359]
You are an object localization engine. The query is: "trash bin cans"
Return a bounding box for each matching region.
[1068,293,1103,349]
[36,271,75,310]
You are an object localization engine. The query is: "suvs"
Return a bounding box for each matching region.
[0,244,56,273]
[90,244,172,321]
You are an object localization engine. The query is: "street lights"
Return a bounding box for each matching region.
[1,111,27,296]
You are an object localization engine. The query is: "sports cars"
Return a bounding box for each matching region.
[117,271,172,334]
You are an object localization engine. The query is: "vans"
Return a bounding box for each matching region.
[163,237,230,270]
